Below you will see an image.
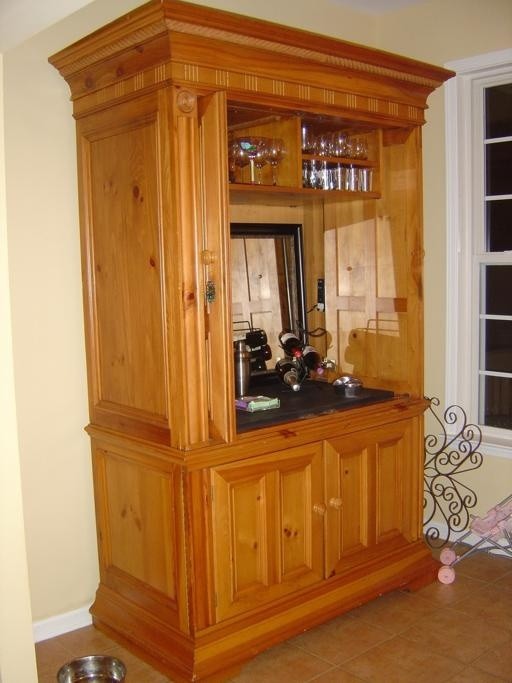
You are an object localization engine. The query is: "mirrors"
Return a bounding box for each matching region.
[227,223,309,390]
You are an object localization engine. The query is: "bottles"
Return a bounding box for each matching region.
[303,160,374,191]
[275,358,301,392]
[302,345,324,375]
[279,329,303,357]
[235,340,250,397]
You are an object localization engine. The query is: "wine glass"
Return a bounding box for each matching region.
[238,137,267,183]
[262,139,288,185]
[232,137,249,183]
[254,137,268,185]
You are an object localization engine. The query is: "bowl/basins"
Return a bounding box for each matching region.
[57,654,126,682]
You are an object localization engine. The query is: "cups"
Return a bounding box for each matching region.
[302,122,368,159]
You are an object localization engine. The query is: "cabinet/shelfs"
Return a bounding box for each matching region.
[46,0,456,683]
